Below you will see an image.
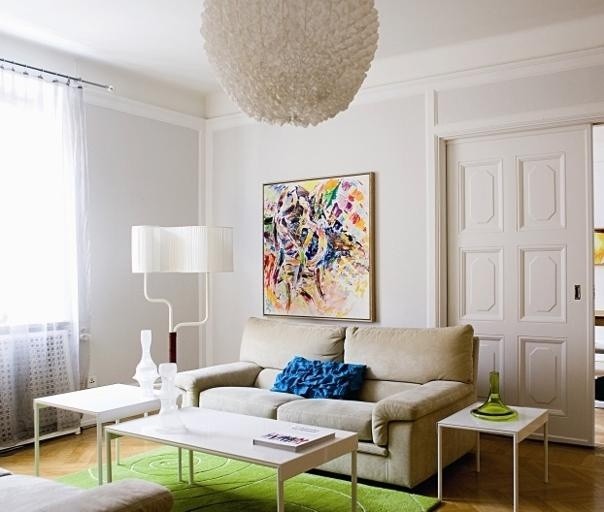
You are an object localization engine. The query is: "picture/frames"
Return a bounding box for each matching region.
[262,172,374,324]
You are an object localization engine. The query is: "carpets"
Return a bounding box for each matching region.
[49,445,442,511]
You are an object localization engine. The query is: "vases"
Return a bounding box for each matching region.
[133,330,160,397]
[152,362,184,429]
[470,371,519,422]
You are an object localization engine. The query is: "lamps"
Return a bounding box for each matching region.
[131,225,233,363]
[200,0,380,127]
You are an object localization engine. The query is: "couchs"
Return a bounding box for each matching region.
[0,466,172,512]
[175,315,479,493]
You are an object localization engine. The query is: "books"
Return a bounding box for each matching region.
[251,424,335,453]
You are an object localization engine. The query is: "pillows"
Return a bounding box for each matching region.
[269,355,366,399]
